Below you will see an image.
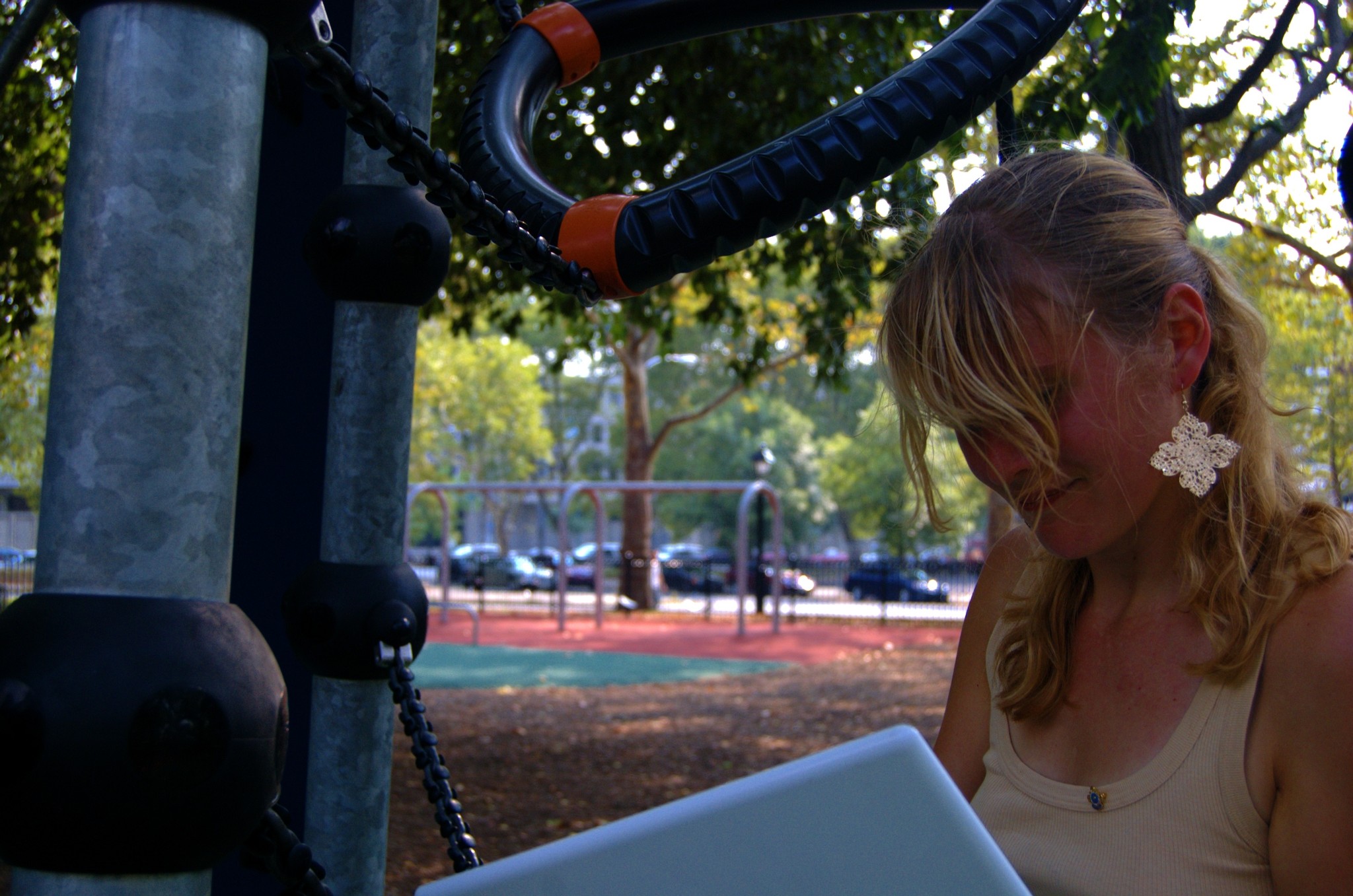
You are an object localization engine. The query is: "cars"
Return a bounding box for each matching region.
[448,542,817,596]
[843,561,947,602]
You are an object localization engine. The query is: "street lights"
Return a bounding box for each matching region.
[744,449,776,612]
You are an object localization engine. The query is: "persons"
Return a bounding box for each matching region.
[878,149,1353,896]
